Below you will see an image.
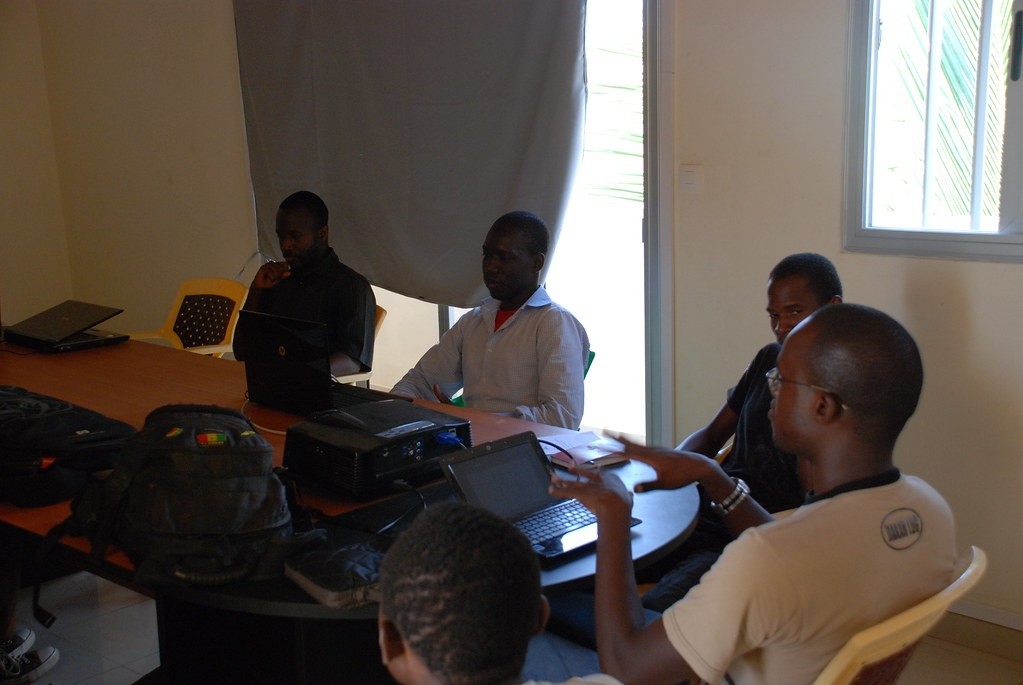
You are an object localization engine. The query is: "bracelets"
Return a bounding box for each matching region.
[711,477,750,516]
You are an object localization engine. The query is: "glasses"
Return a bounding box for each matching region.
[765,366,848,414]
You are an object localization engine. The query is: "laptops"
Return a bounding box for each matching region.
[442,431,643,564]
[2,299,128,354]
[237,309,415,417]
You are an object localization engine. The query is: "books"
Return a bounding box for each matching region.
[537,435,629,470]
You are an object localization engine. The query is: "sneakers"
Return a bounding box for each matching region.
[0,646,62,685]
[0,626,37,658]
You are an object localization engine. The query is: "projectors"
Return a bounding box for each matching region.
[282,397,473,505]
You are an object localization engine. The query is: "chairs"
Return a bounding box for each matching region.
[128,278,249,358]
[332,305,387,389]
[451,350,595,432]
[809,545,988,685]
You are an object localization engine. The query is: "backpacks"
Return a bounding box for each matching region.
[102,401,291,584]
[0,380,138,508]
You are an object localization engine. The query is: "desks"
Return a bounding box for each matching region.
[0,336,700,685]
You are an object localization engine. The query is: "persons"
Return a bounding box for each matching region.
[392,210,590,433]
[0,523,60,685]
[377,499,625,685]
[232,192,374,378]
[639,254,843,613]
[524,303,957,685]
